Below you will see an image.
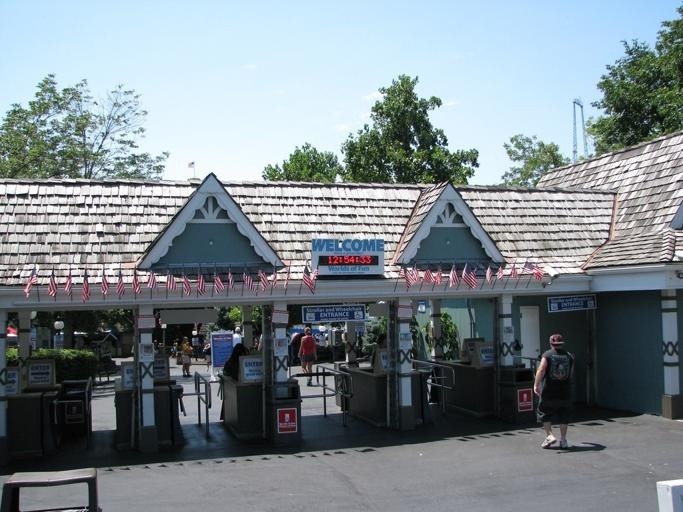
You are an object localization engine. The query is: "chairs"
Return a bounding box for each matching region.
[187,375,192,377]
[307,377,311,386]
[559,439,569,450]
[183,375,187,377]
[542,434,557,449]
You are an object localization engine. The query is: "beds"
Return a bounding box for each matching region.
[202,343,211,352]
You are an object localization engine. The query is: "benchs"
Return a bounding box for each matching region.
[398,258,547,290]
[25,260,321,300]
[412,326,435,427]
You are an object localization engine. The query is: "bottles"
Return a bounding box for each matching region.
[549,334,566,346]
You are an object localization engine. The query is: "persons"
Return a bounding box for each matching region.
[172,339,178,351]
[311,333,329,347]
[180,336,193,377]
[193,345,200,362]
[296,326,317,386]
[205,347,211,364]
[170,343,177,357]
[289,332,304,366]
[153,339,160,350]
[256,333,263,352]
[223,343,248,383]
[183,341,194,358]
[370,333,388,371]
[531,333,578,451]
[233,326,243,347]
